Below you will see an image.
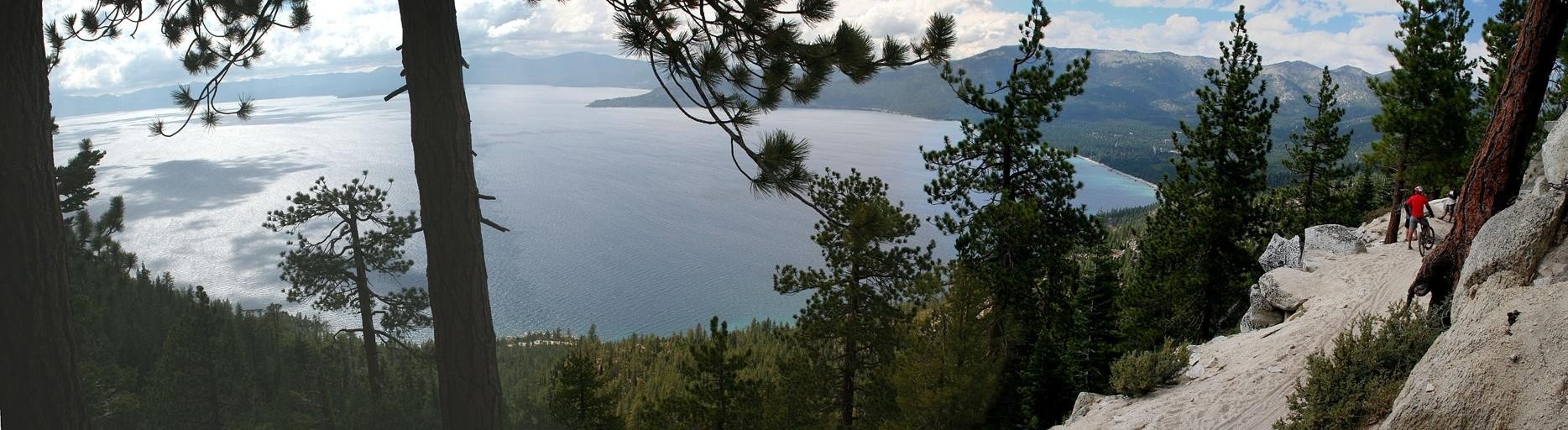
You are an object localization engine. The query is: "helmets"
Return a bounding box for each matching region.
[1415,186,1423,193]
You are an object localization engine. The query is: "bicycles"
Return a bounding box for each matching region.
[1416,215,1434,256]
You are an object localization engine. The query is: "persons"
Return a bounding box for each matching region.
[1405,186,1434,250]
[1438,184,1457,223]
[1403,207,1418,242]
